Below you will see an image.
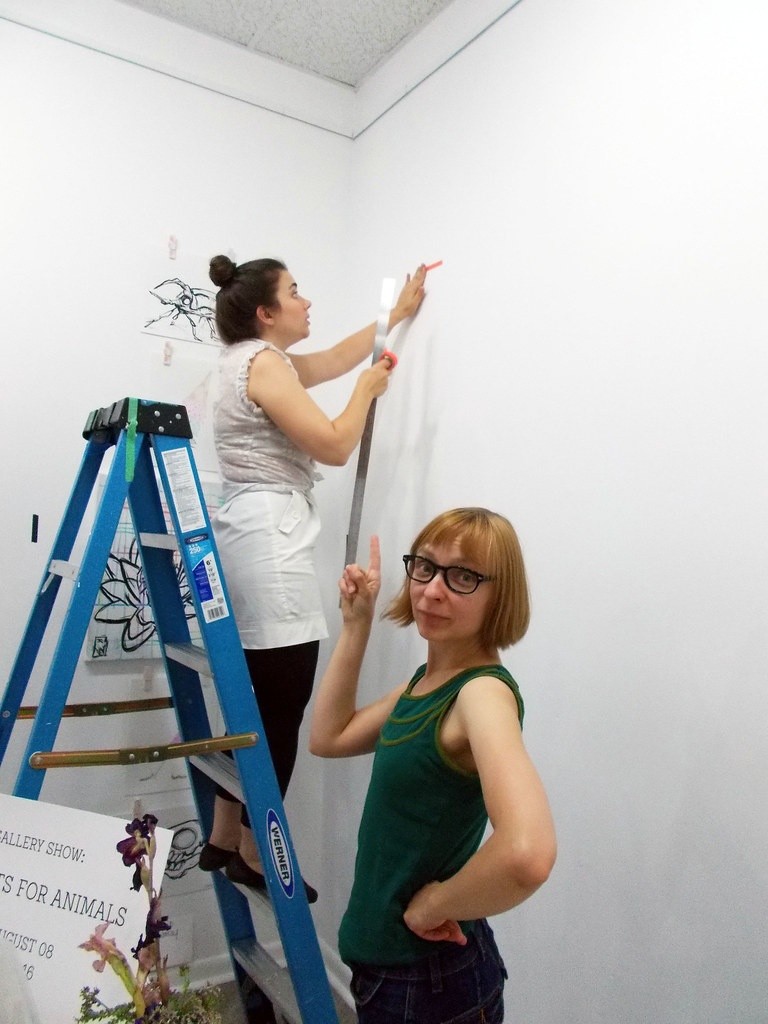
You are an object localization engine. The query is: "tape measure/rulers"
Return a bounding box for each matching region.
[339,276,397,610]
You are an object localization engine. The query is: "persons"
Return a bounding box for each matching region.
[306,507,558,1024]
[195,253,427,904]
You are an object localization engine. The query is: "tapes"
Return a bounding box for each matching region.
[378,350,399,373]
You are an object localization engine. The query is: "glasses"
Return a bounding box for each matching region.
[402,554,494,596]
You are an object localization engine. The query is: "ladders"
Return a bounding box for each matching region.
[0,396,392,1024]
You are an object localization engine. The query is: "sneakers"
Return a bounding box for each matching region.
[199,844,318,902]
